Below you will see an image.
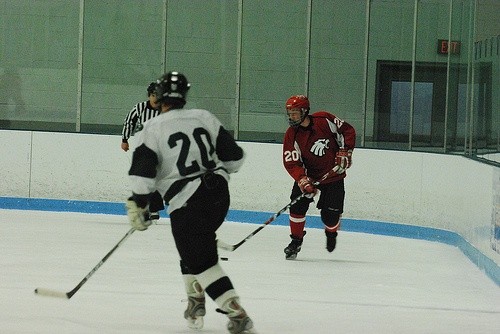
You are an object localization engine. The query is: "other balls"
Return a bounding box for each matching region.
[221,258,228,261]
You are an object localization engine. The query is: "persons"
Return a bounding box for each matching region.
[282,94,356,260]
[125,72,259,334]
[121,81,165,225]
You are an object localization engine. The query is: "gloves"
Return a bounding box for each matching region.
[297,175,320,198]
[125,196,152,231]
[334,149,352,174]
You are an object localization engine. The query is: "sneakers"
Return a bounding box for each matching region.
[149,211,159,224]
[284,231,306,260]
[216,299,255,334]
[324,228,337,252]
[184,293,206,329]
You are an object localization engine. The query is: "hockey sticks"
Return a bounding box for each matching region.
[216,164,341,252]
[34,226,136,300]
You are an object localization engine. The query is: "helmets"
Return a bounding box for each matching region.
[285,95,310,127]
[147,80,160,97]
[154,72,189,105]
